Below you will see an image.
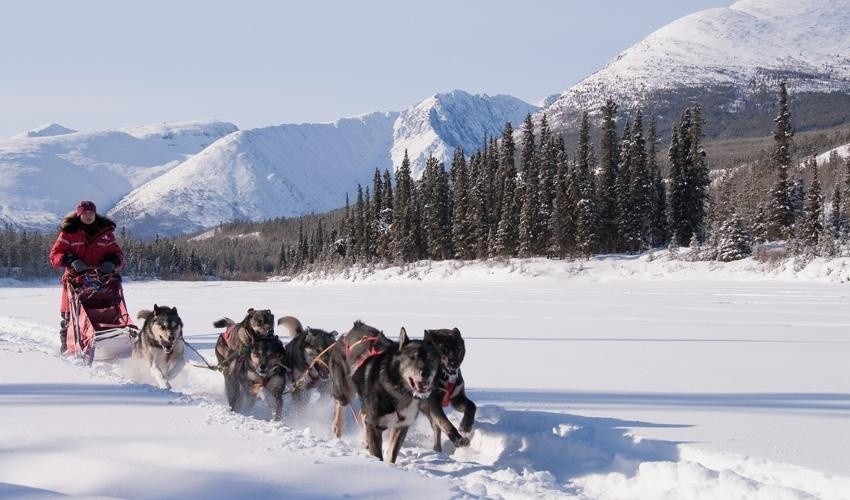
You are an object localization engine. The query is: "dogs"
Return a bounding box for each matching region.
[212,306,277,364]
[417,325,477,460]
[325,325,439,464]
[279,312,339,406]
[221,330,287,422]
[136,302,189,390]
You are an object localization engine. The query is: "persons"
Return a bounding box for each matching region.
[49,201,125,354]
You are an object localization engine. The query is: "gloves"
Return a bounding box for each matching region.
[100,253,120,273]
[62,250,86,273]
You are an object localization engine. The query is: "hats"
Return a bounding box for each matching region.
[76,200,97,217]
